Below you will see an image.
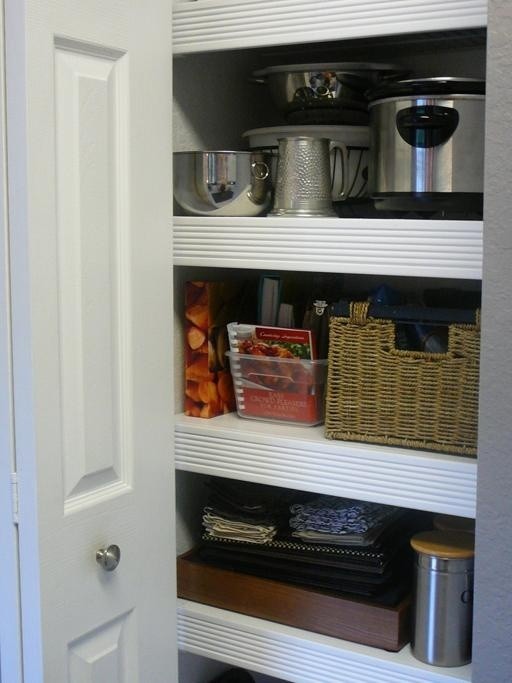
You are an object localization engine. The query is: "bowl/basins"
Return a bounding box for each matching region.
[240,125,370,198]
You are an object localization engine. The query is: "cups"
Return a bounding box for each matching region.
[268,135,348,217]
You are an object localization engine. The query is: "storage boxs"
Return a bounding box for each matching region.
[225,350,327,427]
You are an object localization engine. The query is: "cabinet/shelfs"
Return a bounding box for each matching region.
[170,0,486,683]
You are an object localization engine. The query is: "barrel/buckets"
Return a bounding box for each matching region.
[414,552,475,666]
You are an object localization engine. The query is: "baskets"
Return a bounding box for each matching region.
[324,300,480,457]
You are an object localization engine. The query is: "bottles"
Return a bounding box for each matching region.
[409,529,474,668]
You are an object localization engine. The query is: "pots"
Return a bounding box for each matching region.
[174,149,272,214]
[248,62,417,123]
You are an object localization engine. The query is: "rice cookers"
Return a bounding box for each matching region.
[367,74,484,219]
